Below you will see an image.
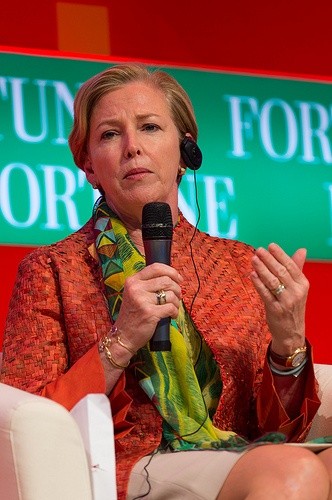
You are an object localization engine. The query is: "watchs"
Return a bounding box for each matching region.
[267,342,308,370]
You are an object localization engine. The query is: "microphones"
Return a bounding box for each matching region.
[142,201,173,353]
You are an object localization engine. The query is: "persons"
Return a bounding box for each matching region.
[0,64,332,500]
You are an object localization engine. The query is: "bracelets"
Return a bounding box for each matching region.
[99,324,139,371]
[271,359,312,377]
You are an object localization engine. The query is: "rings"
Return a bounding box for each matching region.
[157,288,166,305]
[271,283,285,296]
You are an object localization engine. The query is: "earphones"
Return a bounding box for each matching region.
[180,136,202,170]
